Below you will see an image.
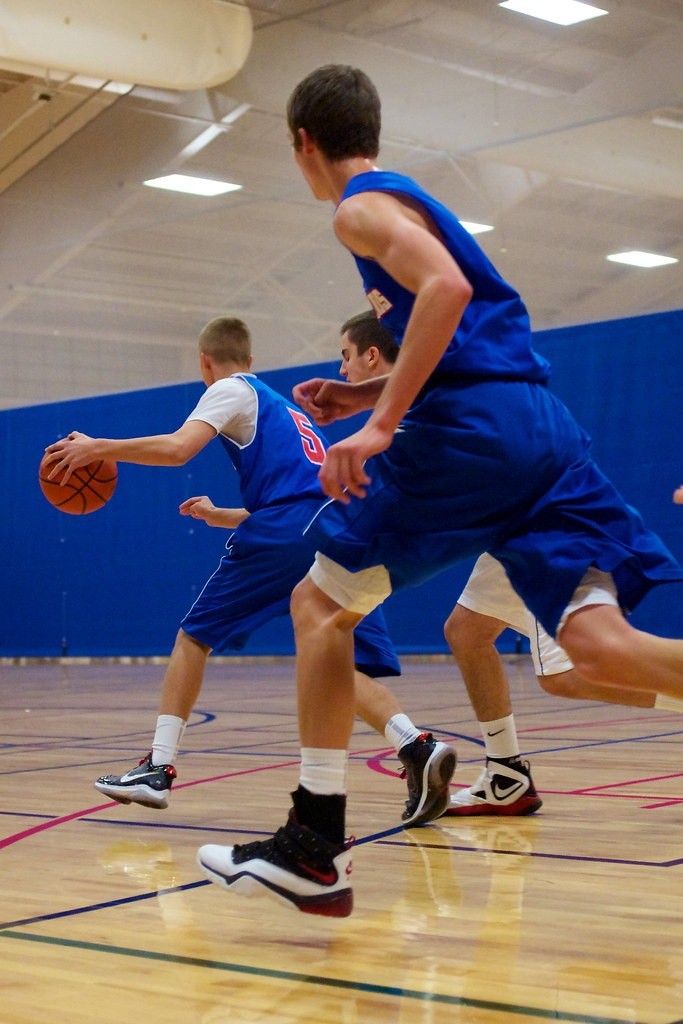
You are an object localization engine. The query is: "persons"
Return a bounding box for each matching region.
[339,309,683,815]
[41,316,458,827]
[196,64,683,915]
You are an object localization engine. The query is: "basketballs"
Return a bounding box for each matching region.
[37,438,120,516]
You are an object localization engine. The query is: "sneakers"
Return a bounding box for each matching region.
[94,748,177,809]
[398,732,457,826]
[197,808,355,919]
[439,762,543,816]
